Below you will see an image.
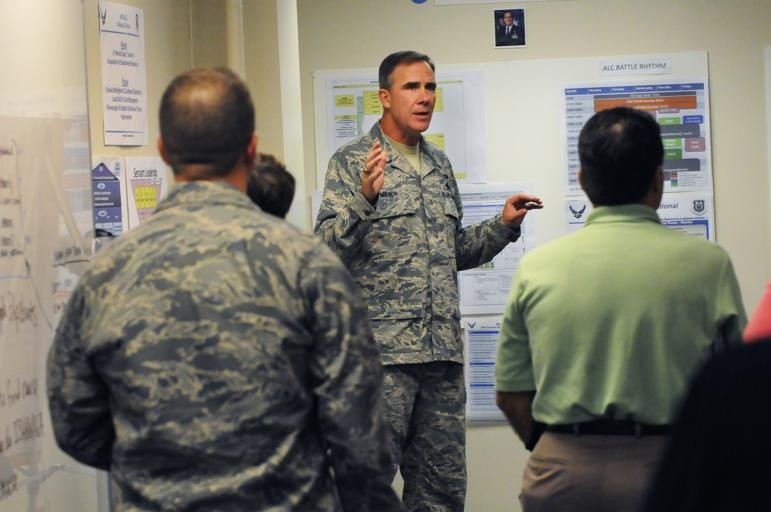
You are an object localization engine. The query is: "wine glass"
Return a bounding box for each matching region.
[364,168,368,173]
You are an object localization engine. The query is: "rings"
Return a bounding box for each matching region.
[364,168,368,173]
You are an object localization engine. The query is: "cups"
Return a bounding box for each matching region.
[503,15,514,18]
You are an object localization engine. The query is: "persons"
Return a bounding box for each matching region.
[314,50,544,512]
[495,107,771,512]
[501,12,520,39]
[46,64,401,512]
[641,293,771,512]
[247,152,296,219]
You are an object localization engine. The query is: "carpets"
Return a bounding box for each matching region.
[506,25,508,34]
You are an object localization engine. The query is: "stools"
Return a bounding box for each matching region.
[538,423,681,436]
